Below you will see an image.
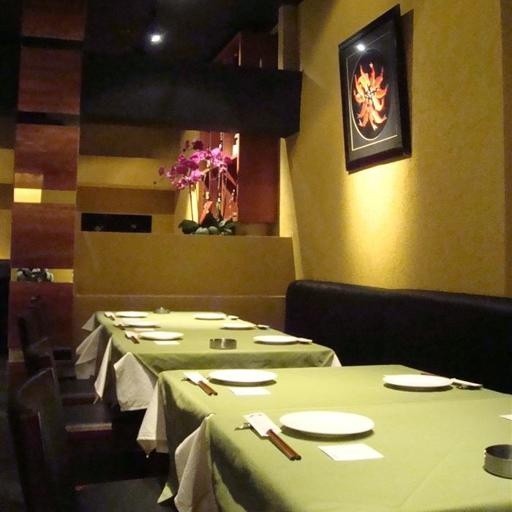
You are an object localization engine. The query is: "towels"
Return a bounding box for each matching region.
[81,313,96,332]
[74,324,103,380]
[173,414,219,512]
[114,351,155,413]
[93,336,111,404]
[136,380,170,459]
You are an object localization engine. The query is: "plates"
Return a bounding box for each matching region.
[280,411,374,436]
[127,319,160,327]
[255,335,296,343]
[210,370,275,384]
[383,374,452,389]
[117,312,145,317]
[195,313,226,320]
[140,331,182,340]
[222,322,255,329]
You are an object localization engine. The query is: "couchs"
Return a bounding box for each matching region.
[286,278,511,392]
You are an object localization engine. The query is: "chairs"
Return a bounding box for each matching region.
[11,362,177,511]
[13,308,112,411]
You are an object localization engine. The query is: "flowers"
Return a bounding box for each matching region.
[158,138,239,235]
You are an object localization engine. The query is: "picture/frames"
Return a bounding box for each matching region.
[338,3,411,170]
[80,213,152,233]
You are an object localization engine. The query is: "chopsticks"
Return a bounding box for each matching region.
[268,431,301,461]
[119,324,124,329]
[131,336,139,344]
[200,382,218,396]
[111,316,115,320]
